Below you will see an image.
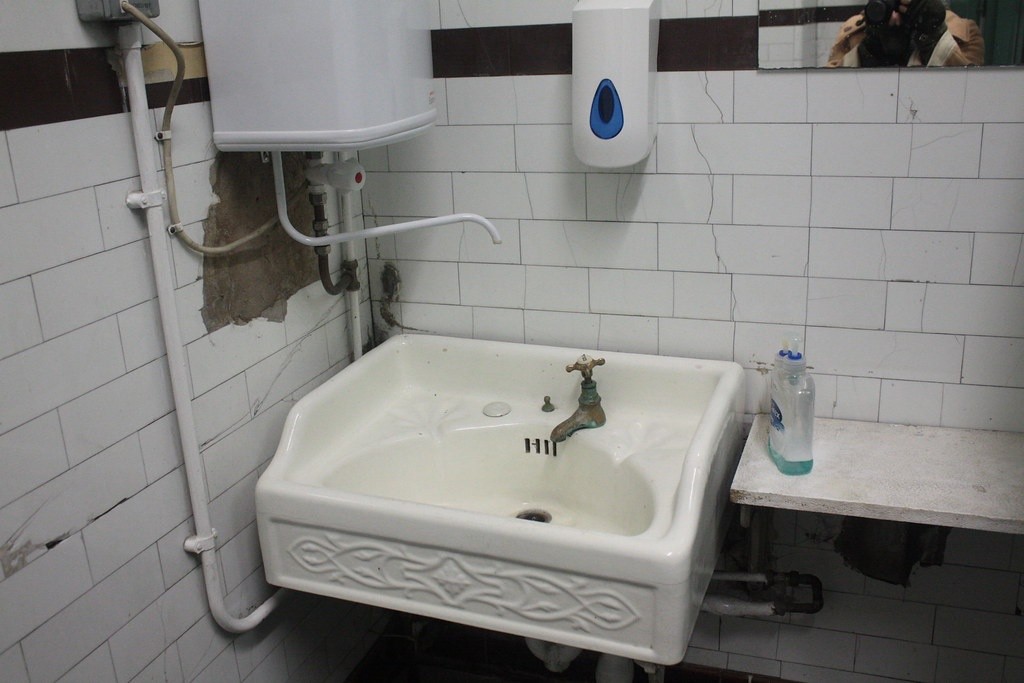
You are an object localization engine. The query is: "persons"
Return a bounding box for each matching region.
[827,0,984,67]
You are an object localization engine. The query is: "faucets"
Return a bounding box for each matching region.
[547,351,607,446]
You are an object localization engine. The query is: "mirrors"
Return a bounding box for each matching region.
[758,1,1024,69]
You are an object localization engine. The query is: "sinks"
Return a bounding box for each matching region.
[249,332,752,670]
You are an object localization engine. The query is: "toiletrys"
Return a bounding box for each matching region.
[766,332,818,477]
[570,0,665,173]
